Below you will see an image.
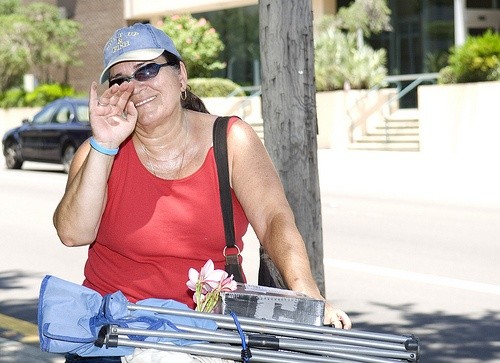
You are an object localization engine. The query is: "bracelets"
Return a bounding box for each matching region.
[89,136,119,157]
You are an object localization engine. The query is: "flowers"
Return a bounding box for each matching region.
[186,259,238,314]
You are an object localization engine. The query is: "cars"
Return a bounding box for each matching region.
[2,98,93,175]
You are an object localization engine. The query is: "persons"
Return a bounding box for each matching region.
[51,23,353,363]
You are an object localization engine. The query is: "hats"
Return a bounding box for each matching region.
[99,23,181,85]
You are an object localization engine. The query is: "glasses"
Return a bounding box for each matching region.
[109,62,174,93]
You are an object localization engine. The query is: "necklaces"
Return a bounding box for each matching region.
[145,112,188,180]
